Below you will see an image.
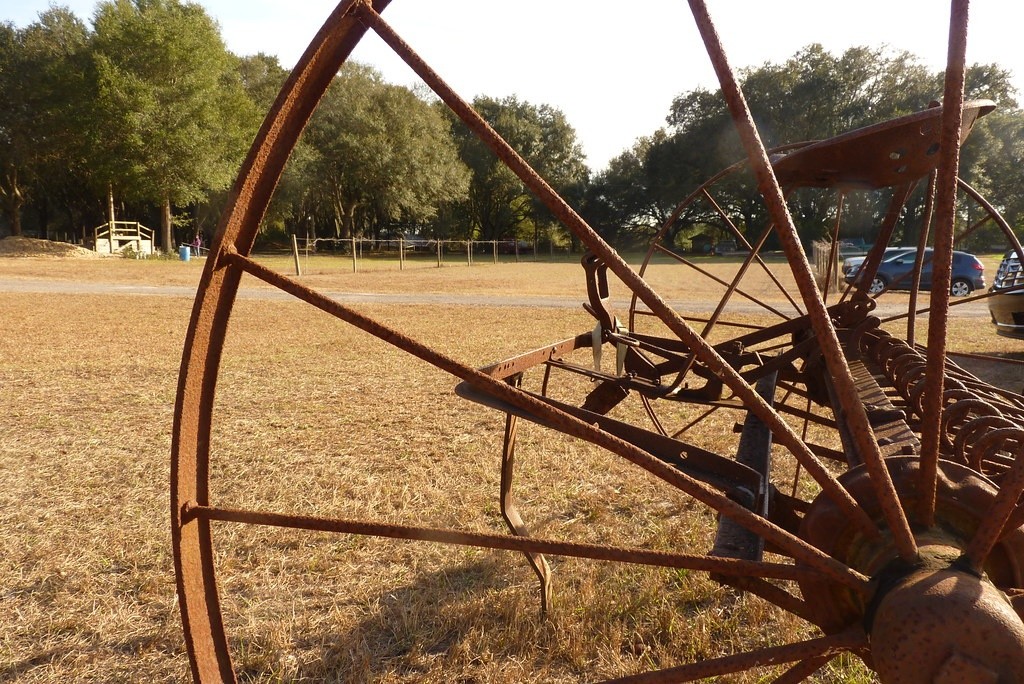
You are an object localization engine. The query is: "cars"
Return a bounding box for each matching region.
[842,245,932,276]
[839,236,869,258]
[847,247,986,296]
[987,245,1024,345]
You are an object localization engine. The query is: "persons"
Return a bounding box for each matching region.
[189,233,208,256]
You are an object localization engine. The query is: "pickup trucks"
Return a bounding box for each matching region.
[714,238,738,257]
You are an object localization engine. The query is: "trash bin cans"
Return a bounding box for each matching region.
[180,246,190,262]
